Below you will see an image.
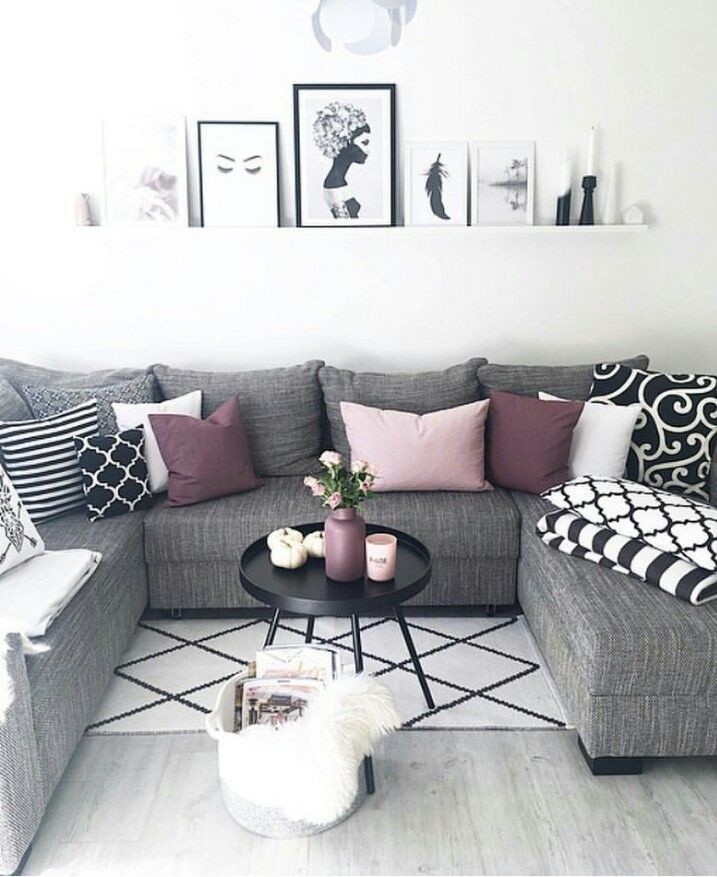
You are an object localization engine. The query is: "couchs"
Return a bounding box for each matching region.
[0,358,717,877]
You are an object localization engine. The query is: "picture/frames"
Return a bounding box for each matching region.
[404,139,469,226]
[470,141,534,225]
[101,115,190,228]
[197,121,280,227]
[293,83,395,228]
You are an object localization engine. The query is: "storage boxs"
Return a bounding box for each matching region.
[205,669,367,839]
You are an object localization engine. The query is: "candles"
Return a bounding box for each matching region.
[557,161,573,196]
[588,126,597,175]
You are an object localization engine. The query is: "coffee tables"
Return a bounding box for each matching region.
[238,521,433,794]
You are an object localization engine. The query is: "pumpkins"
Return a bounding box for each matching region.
[267,527,325,569]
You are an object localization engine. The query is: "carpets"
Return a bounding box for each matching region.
[82,613,576,737]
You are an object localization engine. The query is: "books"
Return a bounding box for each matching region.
[236,644,341,733]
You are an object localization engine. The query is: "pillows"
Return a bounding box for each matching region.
[72,423,155,522]
[111,389,203,494]
[483,389,585,495]
[339,398,495,493]
[148,392,265,508]
[22,375,156,436]
[0,463,45,577]
[586,362,717,502]
[0,398,100,526]
[538,392,644,483]
[539,474,717,571]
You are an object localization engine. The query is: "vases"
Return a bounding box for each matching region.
[325,506,366,581]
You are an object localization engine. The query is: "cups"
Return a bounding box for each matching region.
[365,534,397,581]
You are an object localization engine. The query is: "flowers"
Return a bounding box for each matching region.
[302,450,386,512]
